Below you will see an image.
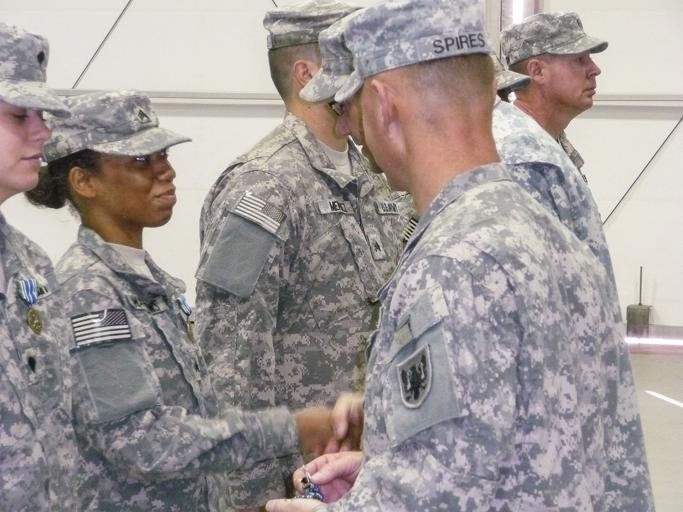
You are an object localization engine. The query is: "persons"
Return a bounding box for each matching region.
[0,19,116,512]
[298,6,616,455]
[191,0,401,512]
[499,12,609,186]
[262,0,656,512]
[24,89,332,512]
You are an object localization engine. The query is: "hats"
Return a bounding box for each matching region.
[497,11,608,66]
[40,87,193,163]
[262,0,489,103]
[0,22,74,118]
[489,52,531,97]
